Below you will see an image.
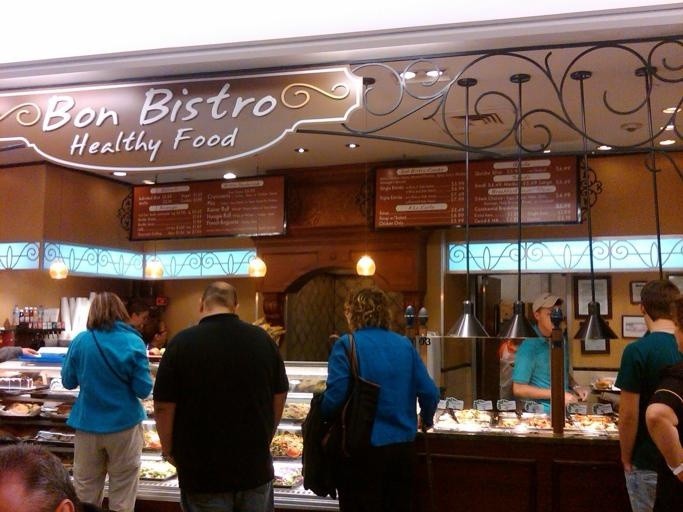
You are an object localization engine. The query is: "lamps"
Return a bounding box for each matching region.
[48,166,270,282]
[570,69,621,339]
[494,72,541,339]
[445,77,492,338]
[354,78,379,277]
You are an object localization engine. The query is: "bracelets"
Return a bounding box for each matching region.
[573,385,581,391]
[666,463,682,476]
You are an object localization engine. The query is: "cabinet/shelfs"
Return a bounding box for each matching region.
[0,346,339,511]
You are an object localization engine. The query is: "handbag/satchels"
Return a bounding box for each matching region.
[299,332,383,502]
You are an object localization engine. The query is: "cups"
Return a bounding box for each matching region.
[60,292,95,341]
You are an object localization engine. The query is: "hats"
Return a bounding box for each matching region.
[531,292,564,315]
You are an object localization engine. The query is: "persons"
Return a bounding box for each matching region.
[645,294,683,512]
[153,280,289,512]
[1,442,84,512]
[0,346,39,363]
[321,285,442,511]
[613,278,680,512]
[510,291,589,415]
[60,291,153,511]
[127,301,151,331]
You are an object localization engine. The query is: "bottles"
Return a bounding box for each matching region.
[11,303,61,329]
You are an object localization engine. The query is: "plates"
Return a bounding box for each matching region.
[147,355,162,361]
